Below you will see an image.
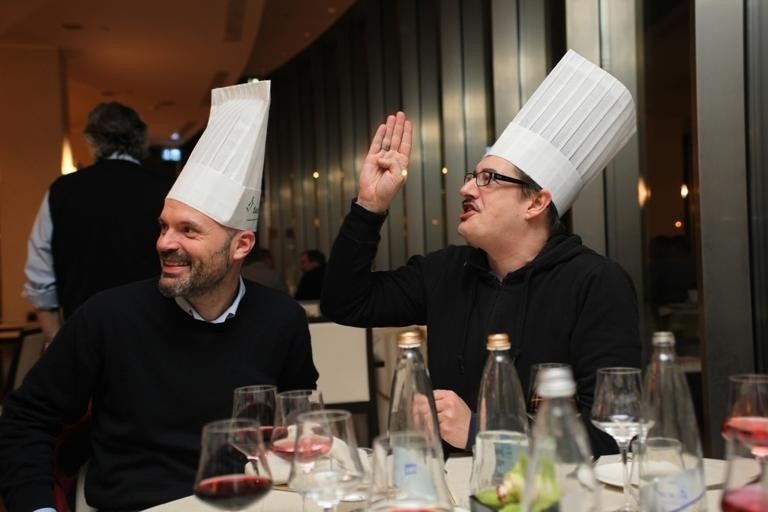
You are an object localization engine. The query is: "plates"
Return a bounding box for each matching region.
[590,459,640,491]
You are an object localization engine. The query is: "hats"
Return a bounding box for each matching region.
[165,80,272,232]
[483,48,638,220]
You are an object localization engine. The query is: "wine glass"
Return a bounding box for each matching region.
[589,369,651,509]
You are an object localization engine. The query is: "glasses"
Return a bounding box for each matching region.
[464,170,529,187]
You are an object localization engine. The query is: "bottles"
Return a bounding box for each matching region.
[385,328,455,509]
[719,372,768,512]
[626,331,707,511]
[474,332,530,434]
[528,377,601,508]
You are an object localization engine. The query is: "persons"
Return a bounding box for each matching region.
[294,250,327,301]
[22,101,175,511]
[1,197,319,511]
[320,156,644,461]
[241,247,288,294]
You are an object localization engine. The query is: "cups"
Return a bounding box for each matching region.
[229,386,287,479]
[629,436,686,498]
[196,419,274,510]
[328,446,376,511]
[287,409,366,511]
[467,429,560,512]
[268,388,334,485]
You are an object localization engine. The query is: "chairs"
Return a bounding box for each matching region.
[307,316,379,450]
[0,327,45,393]
